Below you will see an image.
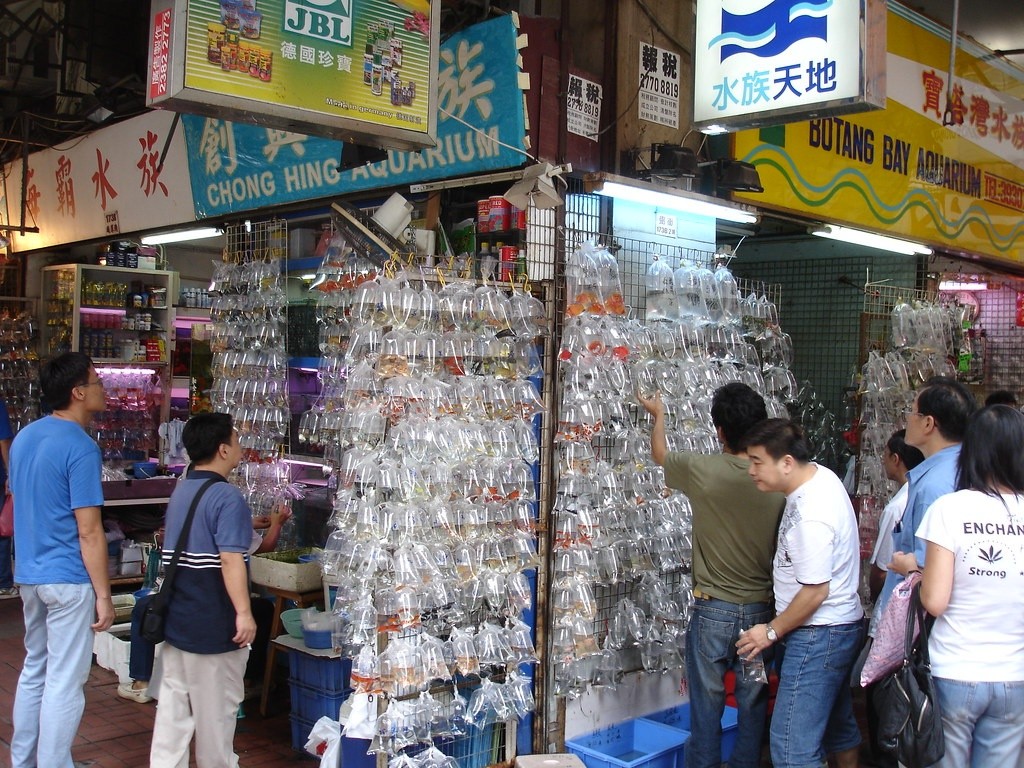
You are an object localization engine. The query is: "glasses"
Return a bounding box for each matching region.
[73,377,103,390]
[901,406,938,428]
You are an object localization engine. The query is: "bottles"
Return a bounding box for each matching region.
[476,242,504,281]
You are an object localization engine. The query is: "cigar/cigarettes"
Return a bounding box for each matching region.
[247,643,253,651]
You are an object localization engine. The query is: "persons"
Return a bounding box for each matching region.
[886,404,1024,768]
[637,382,778,768]
[868,428,927,613]
[10,351,116,768]
[983,390,1024,414]
[149,409,257,768]
[0,389,21,599]
[867,376,978,768]
[117,502,293,704]
[735,418,866,768]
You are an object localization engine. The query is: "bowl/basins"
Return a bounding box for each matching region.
[298,554,313,564]
[300,625,336,649]
[280,607,321,639]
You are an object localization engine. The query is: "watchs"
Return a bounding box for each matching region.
[765,622,777,642]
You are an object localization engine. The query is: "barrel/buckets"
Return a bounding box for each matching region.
[132,462,160,479]
[133,588,151,603]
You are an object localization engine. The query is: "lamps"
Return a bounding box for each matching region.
[582,171,761,225]
[807,223,934,256]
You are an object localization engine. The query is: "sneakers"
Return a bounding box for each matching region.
[118,683,154,703]
[0,586,22,599]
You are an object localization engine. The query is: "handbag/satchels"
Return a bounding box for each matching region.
[876,583,944,768]
[861,572,928,688]
[138,594,165,643]
[0,493,14,536]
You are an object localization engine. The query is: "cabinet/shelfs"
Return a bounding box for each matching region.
[166,191,546,540]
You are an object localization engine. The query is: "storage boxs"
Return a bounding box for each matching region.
[89,543,739,768]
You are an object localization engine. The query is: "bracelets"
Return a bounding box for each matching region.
[907,570,922,574]
